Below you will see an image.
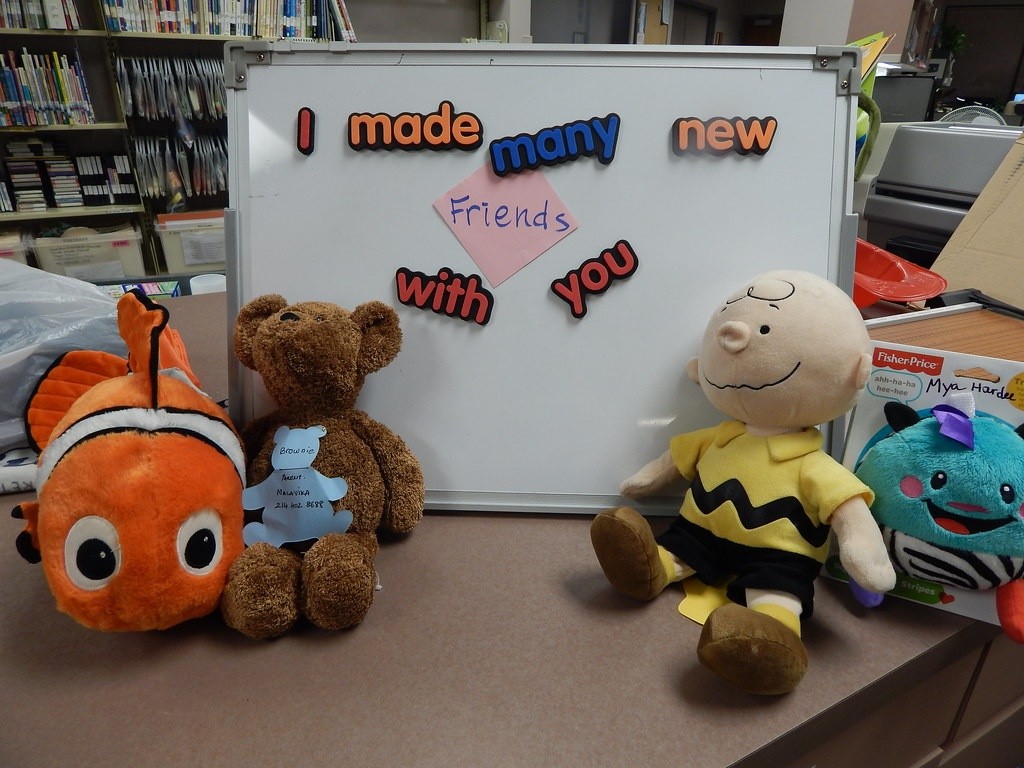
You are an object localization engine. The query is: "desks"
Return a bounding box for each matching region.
[0,292,1024,768]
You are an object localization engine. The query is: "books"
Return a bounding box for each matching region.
[0,151,140,213]
[100,0,357,43]
[157,209,226,228]
[0,44,95,126]
[0,0,84,29]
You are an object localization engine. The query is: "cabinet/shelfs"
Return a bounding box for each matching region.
[0,0,318,286]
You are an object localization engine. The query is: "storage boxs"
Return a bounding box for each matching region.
[0,231,28,265]
[25,222,146,280]
[154,219,224,274]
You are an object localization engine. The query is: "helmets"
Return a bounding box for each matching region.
[853,238,947,310]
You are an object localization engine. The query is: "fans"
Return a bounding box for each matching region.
[939,105,1007,126]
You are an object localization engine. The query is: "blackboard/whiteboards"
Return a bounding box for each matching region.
[223,39,863,517]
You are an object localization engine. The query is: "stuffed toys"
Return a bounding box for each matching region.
[8,286,250,633]
[221,294,425,644]
[846,391,1024,645]
[592,271,895,699]
